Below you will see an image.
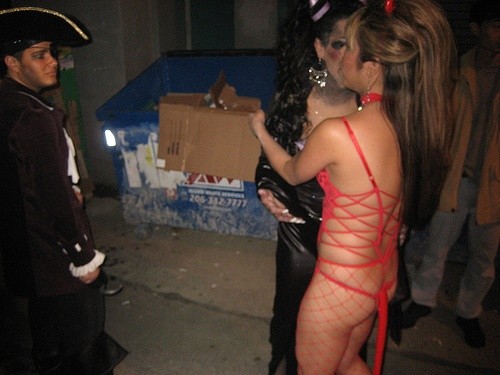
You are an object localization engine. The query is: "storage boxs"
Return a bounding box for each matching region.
[155,71,261,182]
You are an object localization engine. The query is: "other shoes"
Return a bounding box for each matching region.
[454,314,485,348]
[399,300,433,328]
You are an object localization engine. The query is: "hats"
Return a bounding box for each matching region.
[0,6,92,50]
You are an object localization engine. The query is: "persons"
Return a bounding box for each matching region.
[246,0,458,375]
[400,0,500,348]
[0,7,129,375]
